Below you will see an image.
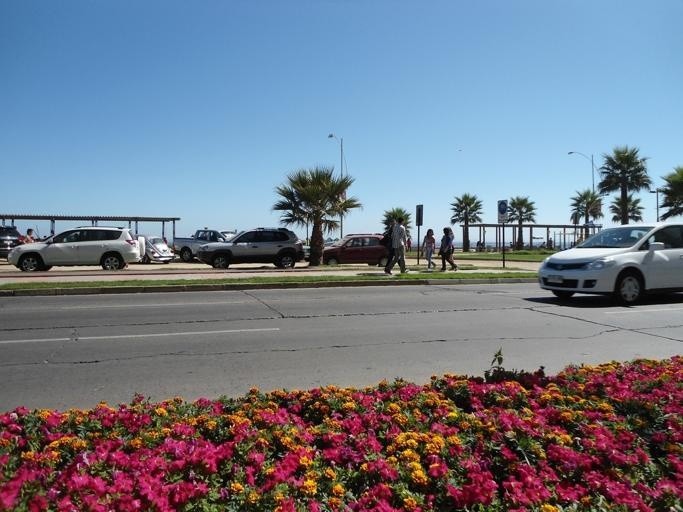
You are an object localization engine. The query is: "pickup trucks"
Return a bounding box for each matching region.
[172,224,229,262]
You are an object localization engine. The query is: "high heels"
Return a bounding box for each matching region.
[450,264,457,271]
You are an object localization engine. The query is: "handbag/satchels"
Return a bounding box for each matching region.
[379,233,392,248]
[447,248,453,254]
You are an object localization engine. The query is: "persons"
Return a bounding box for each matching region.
[407,238,412,252]
[421,226,438,269]
[382,216,397,275]
[448,227,455,255]
[439,226,457,271]
[388,219,410,275]
[477,238,563,253]
[23,228,37,244]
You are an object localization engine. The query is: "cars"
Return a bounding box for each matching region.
[146,233,174,263]
[220,230,237,239]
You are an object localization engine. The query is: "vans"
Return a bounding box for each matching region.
[538,222,682,304]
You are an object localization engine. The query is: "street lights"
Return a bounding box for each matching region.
[648,188,660,222]
[328,133,344,239]
[566,151,594,211]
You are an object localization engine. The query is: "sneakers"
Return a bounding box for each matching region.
[385,268,391,275]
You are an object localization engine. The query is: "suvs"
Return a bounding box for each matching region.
[303,232,390,268]
[196,228,304,268]
[0,226,21,258]
[6,225,146,271]
